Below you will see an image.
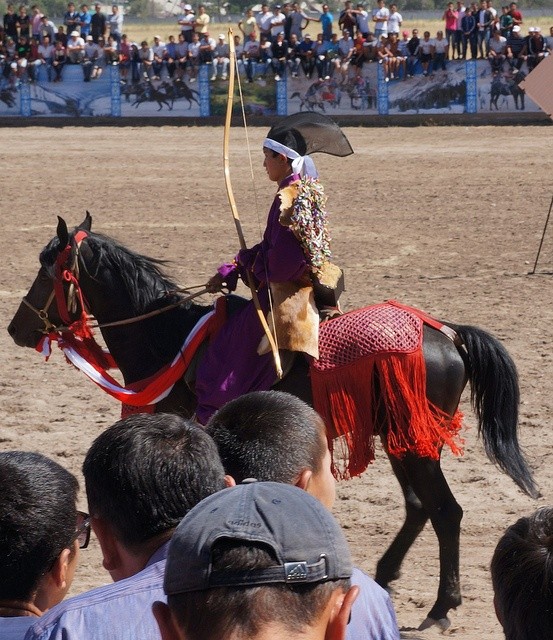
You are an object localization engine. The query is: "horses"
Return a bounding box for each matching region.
[7,211,539,634]
[118,78,200,110]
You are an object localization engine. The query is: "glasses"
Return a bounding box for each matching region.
[70,511,91,550]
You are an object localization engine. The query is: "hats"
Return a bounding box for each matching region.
[162,479,351,595]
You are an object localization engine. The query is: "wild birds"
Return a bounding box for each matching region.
[289,81,324,113]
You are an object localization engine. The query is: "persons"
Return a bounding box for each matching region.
[0,449,93,639]
[488,505,552,640]
[22,409,405,639]
[183,108,356,444]
[148,477,362,640]
[205,387,344,523]
